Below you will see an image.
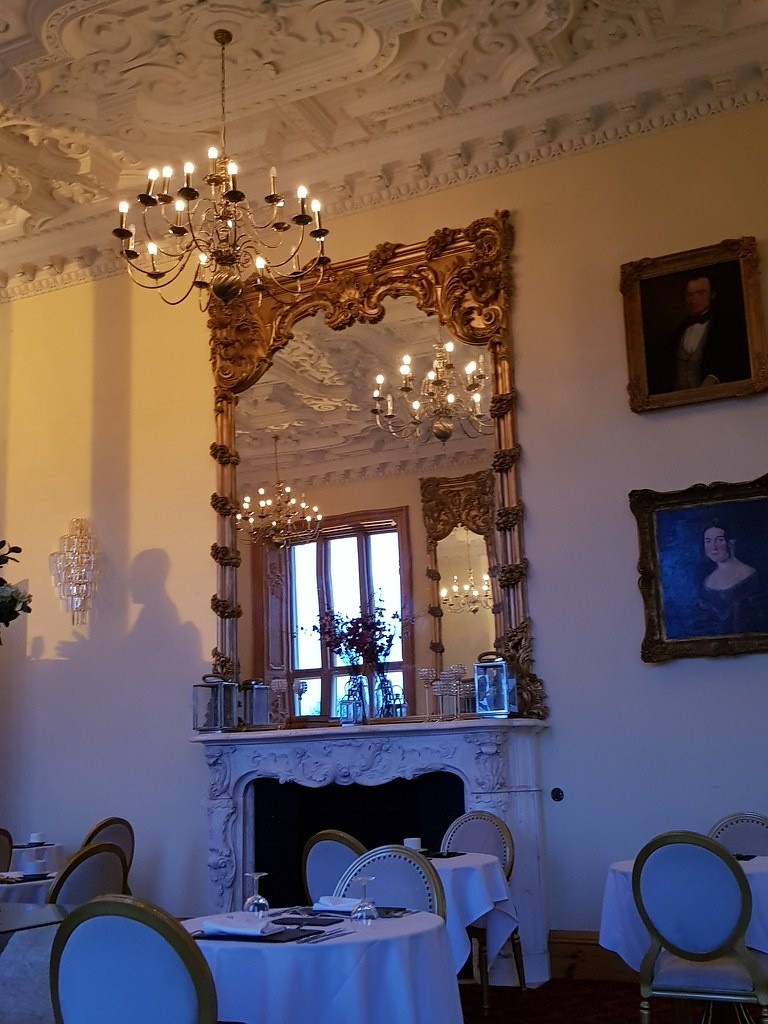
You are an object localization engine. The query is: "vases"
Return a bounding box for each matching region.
[372,676,396,718]
[338,675,365,725]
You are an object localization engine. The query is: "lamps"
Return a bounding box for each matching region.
[48,517,98,627]
[237,431,325,552]
[370,315,494,446]
[113,28,332,317]
[441,527,494,617]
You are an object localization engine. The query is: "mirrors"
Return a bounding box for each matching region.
[208,211,552,722]
[418,478,500,692]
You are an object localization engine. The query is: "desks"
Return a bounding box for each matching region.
[425,851,520,1008]
[0,903,80,955]
[10,843,67,872]
[599,857,768,1024]
[0,870,56,904]
[178,905,464,1024]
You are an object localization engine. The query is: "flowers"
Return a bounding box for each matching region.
[349,589,401,712]
[301,600,372,714]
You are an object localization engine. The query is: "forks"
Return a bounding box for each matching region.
[294,909,352,918]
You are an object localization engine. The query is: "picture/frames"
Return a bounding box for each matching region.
[628,472,768,662]
[619,235,768,411]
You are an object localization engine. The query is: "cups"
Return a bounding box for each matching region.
[30,833,44,842]
[25,860,47,874]
[404,837,421,850]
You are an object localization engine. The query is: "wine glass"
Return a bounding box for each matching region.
[352,875,378,928]
[244,873,269,922]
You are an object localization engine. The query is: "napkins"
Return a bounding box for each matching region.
[313,896,361,911]
[204,915,285,937]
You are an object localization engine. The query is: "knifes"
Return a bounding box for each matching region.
[297,927,355,943]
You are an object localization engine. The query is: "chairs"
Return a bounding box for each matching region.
[0,813,768,1024]
